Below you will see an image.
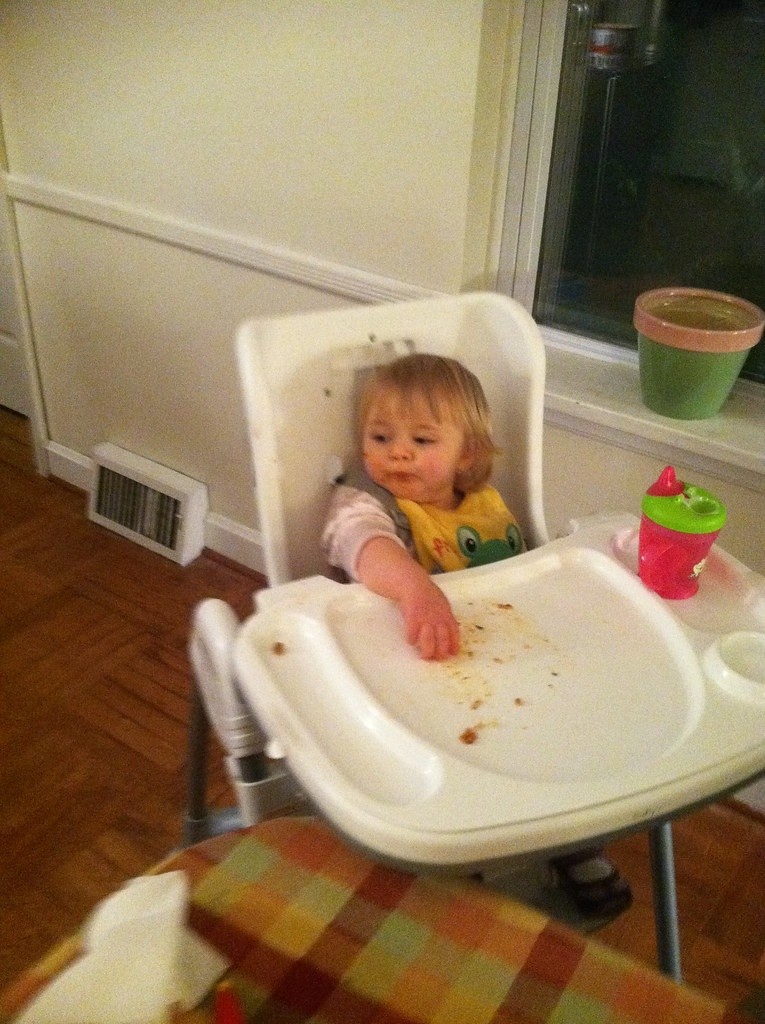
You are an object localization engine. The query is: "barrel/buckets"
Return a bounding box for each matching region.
[632,286,765,422]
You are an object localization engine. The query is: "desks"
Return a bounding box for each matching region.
[0,818,765,1024]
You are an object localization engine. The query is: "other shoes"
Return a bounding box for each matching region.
[551,849,632,920]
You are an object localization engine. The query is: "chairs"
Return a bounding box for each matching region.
[185,289,685,982]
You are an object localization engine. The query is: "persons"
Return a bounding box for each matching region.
[322,355,634,916]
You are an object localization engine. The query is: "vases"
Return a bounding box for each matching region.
[633,286,765,422]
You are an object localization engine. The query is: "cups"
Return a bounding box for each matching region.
[637,465,727,599]
